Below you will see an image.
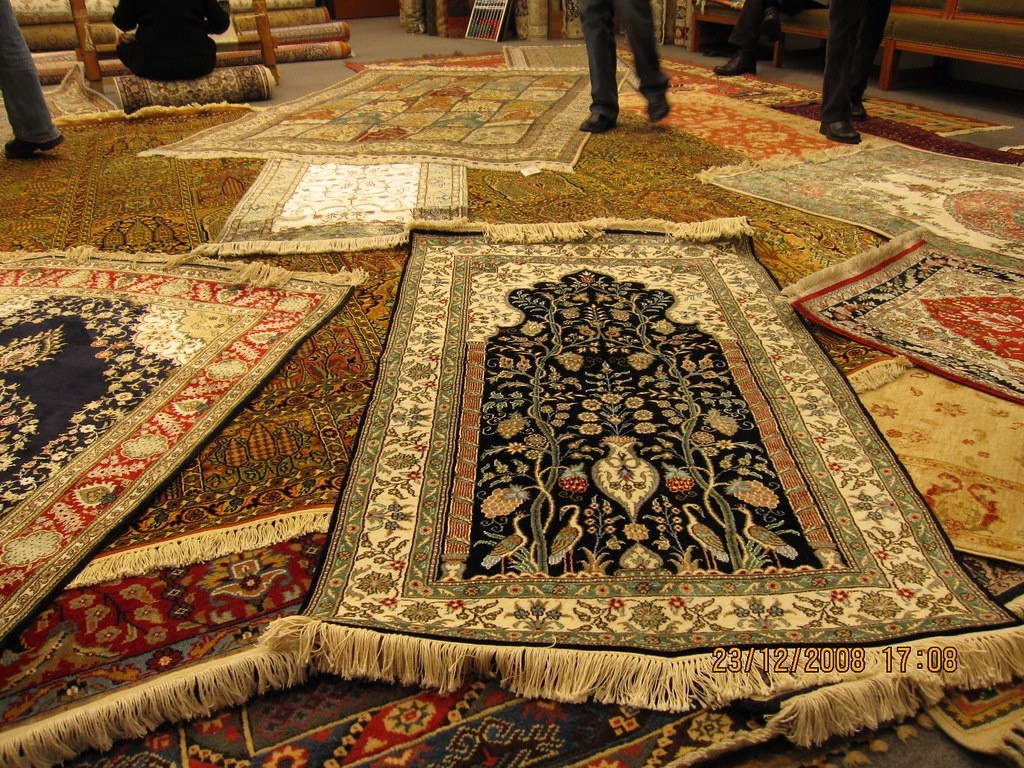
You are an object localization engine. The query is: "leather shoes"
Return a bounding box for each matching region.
[762,13,781,43]
[819,121,860,144]
[850,100,867,121]
[648,94,669,121]
[579,113,617,133]
[714,56,756,75]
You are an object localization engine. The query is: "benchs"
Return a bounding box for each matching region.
[690,0,1024,92]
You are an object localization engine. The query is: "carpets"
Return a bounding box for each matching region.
[0,0,1024,768]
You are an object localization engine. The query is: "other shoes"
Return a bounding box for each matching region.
[5,134,66,156]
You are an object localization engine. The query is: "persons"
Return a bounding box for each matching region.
[576,1,670,135]
[110,0,233,84]
[0,1,62,161]
[713,2,893,147]
